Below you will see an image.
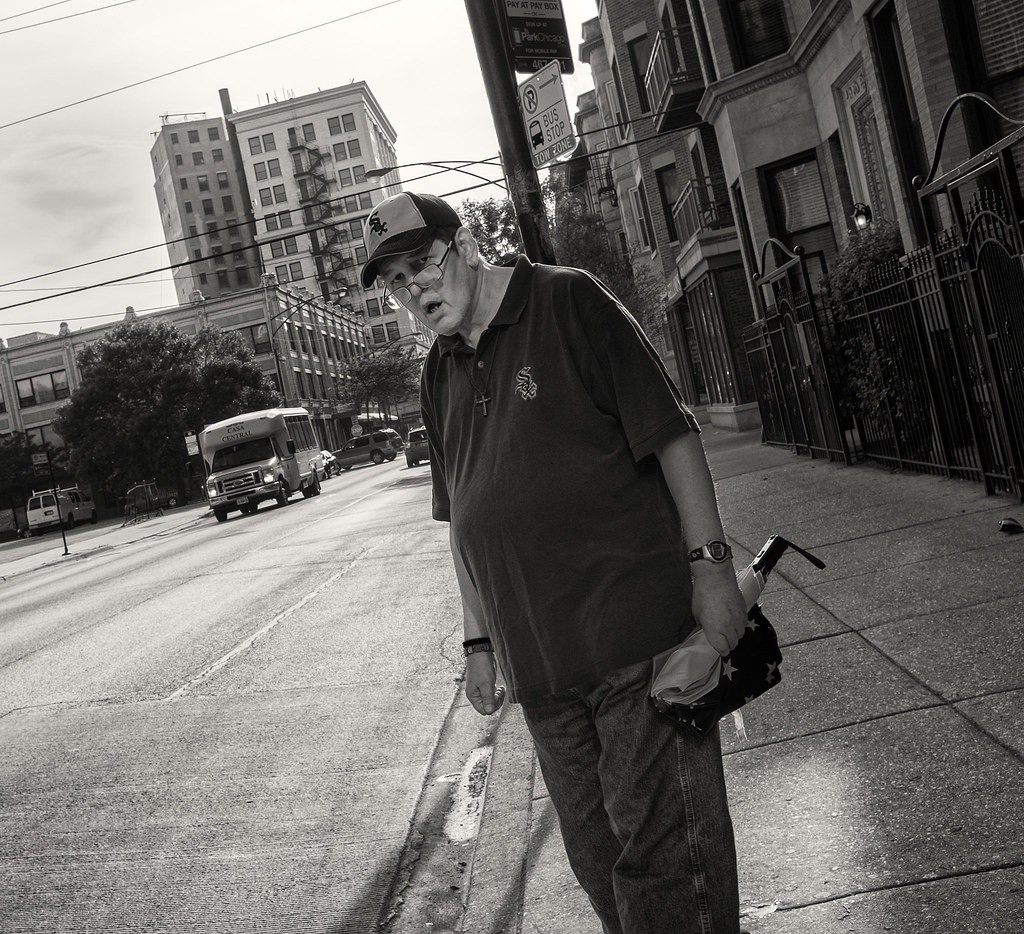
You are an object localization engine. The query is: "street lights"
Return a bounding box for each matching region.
[364,163,558,265]
[266,287,348,409]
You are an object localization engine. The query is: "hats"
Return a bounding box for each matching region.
[359,191,462,288]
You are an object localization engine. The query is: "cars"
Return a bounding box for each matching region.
[119,485,181,516]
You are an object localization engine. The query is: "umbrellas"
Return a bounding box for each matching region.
[651,534,825,736]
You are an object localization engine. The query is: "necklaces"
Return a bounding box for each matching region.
[461,326,502,417]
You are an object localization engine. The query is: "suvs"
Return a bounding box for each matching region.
[404,426,428,470]
[330,429,406,470]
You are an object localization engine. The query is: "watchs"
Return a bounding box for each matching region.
[687,538,734,565]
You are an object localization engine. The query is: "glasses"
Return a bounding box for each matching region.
[382,232,458,312]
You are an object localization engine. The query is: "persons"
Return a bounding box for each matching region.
[360,191,829,934]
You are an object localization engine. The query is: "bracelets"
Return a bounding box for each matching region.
[462,637,495,657]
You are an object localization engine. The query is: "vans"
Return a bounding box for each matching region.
[26,489,97,535]
[197,406,326,522]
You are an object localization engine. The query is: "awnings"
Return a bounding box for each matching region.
[358,412,398,424]
